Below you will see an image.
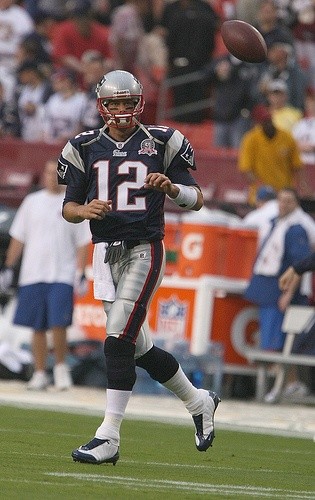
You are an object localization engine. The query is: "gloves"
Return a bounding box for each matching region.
[104,241,127,265]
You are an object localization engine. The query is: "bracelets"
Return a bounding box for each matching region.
[167,184,198,208]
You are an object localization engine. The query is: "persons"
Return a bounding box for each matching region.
[0,0,315,163]
[57,69,220,465]
[0,159,90,391]
[238,105,302,195]
[244,188,315,400]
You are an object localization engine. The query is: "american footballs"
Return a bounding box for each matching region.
[221,19,267,64]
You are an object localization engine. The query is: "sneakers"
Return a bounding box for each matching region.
[71,438,120,465]
[191,391,222,452]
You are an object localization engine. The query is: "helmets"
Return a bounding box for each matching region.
[95,70,143,126]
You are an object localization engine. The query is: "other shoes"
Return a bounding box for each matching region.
[26,373,49,391]
[53,365,71,391]
